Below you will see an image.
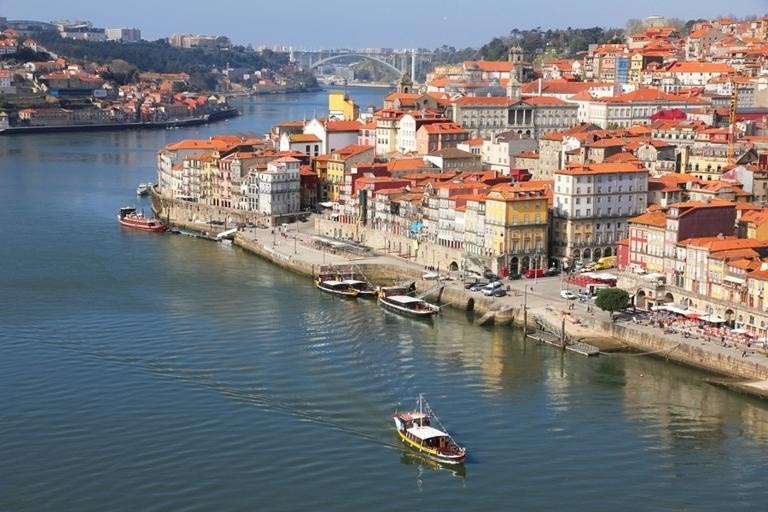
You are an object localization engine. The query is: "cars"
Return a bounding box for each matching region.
[576,262,596,273]
[465,274,506,297]
[544,267,559,276]
[561,290,575,299]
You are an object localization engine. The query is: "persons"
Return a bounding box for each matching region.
[634,304,764,356]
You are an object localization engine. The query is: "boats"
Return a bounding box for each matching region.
[117,205,167,231]
[137,184,147,196]
[392,393,465,464]
[379,285,435,314]
[315,272,376,296]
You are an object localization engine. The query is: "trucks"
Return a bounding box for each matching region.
[595,256,617,270]
[526,269,543,278]
